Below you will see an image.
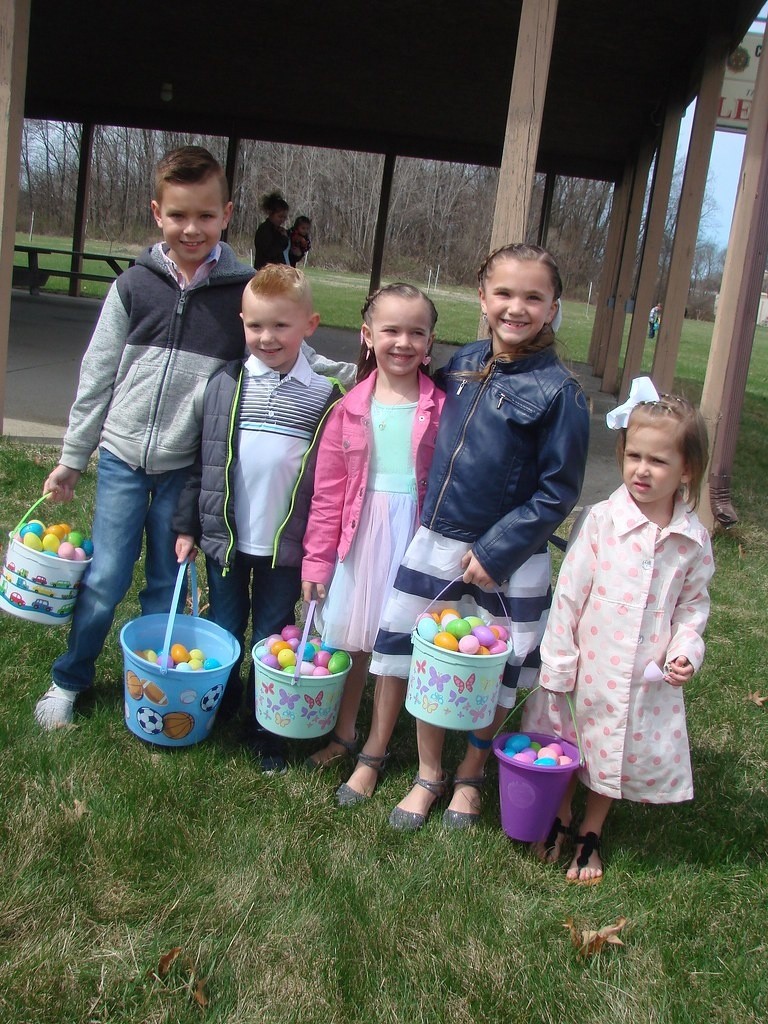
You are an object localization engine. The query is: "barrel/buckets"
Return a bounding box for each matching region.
[120,556,242,746]
[0,493,94,625]
[251,600,352,738]
[490,686,586,842]
[404,575,514,731]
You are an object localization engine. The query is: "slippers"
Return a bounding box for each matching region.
[536,818,573,867]
[564,830,603,886]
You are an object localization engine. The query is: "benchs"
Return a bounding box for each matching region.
[12,265,123,297]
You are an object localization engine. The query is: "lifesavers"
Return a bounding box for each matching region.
[290,234,308,252]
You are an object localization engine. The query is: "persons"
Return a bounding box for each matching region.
[171,263,347,774]
[520,391,716,885]
[370,243,591,830]
[302,283,446,807]
[288,216,310,267]
[648,303,663,338]
[33,145,361,736]
[253,193,291,270]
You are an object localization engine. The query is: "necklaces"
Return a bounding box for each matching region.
[372,387,411,430]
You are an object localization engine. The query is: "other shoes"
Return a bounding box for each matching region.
[389,769,449,832]
[335,747,391,808]
[303,730,358,775]
[442,770,489,829]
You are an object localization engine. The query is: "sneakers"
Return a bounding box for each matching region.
[35,680,80,733]
[216,703,238,723]
[257,743,288,777]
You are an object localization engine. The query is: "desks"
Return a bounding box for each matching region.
[13,242,137,304]
